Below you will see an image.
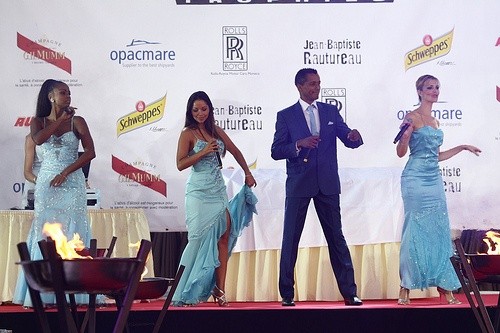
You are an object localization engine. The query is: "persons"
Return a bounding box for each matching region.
[271,68,363,306]
[169,91,258,306]
[14,79,108,305]
[397,75,482,306]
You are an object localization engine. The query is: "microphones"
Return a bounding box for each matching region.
[63,106,73,114]
[212,141,222,166]
[304,140,316,163]
[393,118,412,144]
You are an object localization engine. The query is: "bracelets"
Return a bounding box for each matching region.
[246,173,252,177]
[33,176,37,184]
[60,173,66,177]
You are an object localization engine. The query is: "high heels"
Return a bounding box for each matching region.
[436,286,461,305]
[177,300,192,307]
[397,287,410,305]
[210,286,232,307]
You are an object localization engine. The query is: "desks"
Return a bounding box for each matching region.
[221,166,440,303]
[0,209,155,305]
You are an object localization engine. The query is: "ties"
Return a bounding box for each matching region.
[307,105,319,137]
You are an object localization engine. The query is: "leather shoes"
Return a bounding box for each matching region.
[281,297,295,306]
[344,296,363,305]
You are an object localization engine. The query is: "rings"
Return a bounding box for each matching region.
[55,183,58,186]
